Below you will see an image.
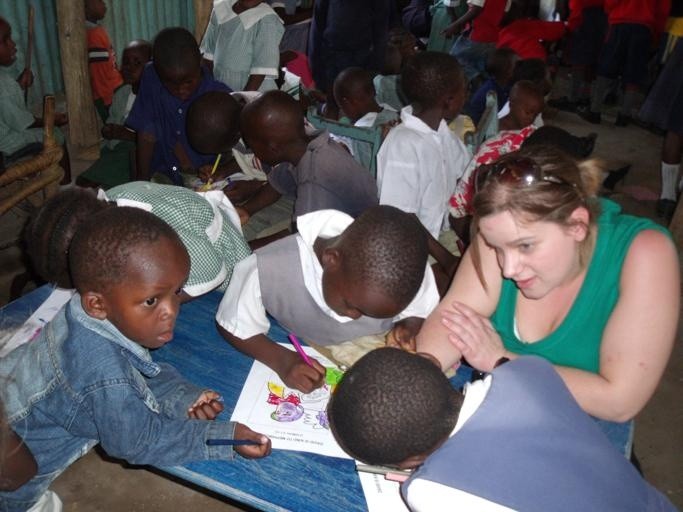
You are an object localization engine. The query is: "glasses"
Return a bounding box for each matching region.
[473,156,574,192]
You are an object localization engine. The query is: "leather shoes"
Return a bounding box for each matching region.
[547,95,631,190]
[655,187,681,226]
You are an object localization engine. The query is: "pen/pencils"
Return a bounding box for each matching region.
[288,333,331,393]
[455,238,465,257]
[227,176,232,190]
[206,438,256,448]
[206,154,222,186]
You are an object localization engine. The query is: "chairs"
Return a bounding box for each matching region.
[302,109,382,182]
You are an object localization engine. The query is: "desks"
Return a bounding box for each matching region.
[0,284,481,511]
